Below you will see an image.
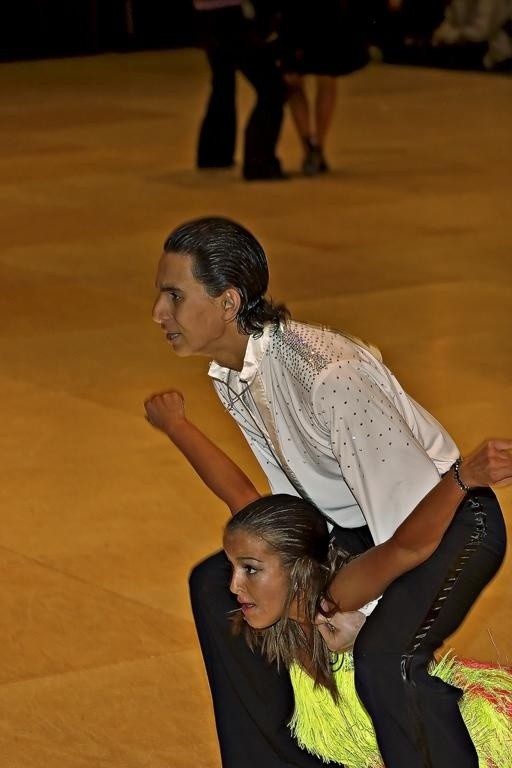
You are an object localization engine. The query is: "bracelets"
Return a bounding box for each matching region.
[453,458,470,492]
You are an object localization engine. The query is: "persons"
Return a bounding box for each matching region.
[192,0,373,180]
[151,216,506,766]
[142,390,511,767]
[369,1,511,74]
